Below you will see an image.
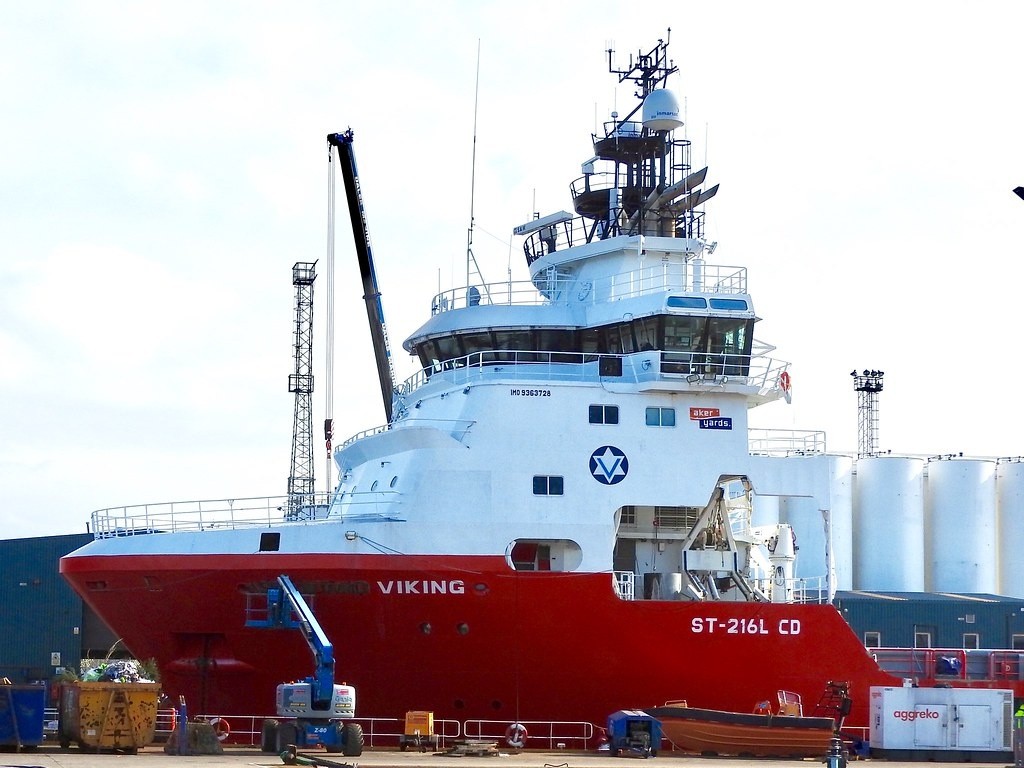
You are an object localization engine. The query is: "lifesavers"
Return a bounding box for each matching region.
[505,724,529,747]
[780,371,790,391]
[209,717,231,741]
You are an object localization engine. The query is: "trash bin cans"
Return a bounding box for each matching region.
[57,680,162,755]
[0,685,48,752]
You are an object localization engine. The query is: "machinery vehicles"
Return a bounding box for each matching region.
[318,124,393,507]
[243,572,366,756]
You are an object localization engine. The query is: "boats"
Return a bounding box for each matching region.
[55,21,1024,747]
[644,680,852,760]
